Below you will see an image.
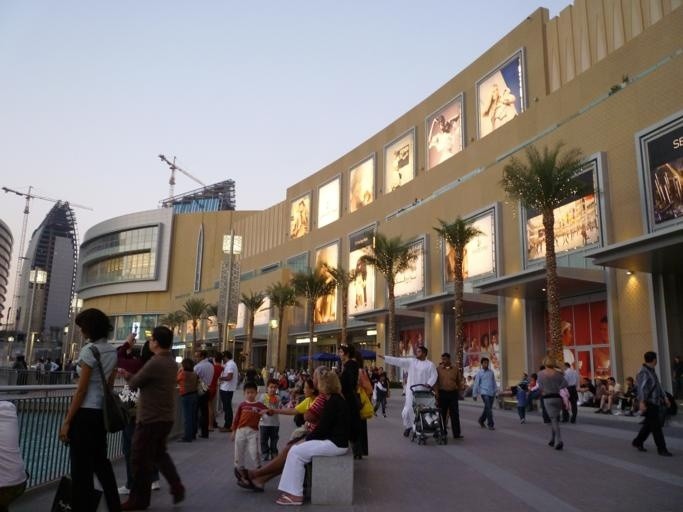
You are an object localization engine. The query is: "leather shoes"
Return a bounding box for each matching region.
[658,451,672,456]
[632,441,647,452]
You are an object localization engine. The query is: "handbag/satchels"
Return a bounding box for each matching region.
[357,386,374,419]
[196,376,209,395]
[103,388,129,432]
[51,475,102,512]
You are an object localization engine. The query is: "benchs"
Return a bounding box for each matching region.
[310,441,355,505]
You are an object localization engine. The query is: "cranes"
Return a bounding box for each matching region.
[156,152,206,198]
[1,184,95,330]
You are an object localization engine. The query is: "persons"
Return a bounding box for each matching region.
[228,380,273,472]
[271,367,350,506]
[120,326,187,511]
[0,398,30,511]
[436,352,683,457]
[10,352,60,391]
[396,332,425,382]
[174,355,197,443]
[193,350,214,440]
[63,358,73,384]
[427,113,461,166]
[462,328,503,397]
[560,320,575,371]
[112,331,163,496]
[480,82,517,131]
[258,379,318,439]
[233,364,329,492]
[593,315,611,376]
[257,378,282,460]
[242,365,391,419]
[56,306,122,512]
[218,350,238,432]
[207,351,223,434]
[376,345,439,439]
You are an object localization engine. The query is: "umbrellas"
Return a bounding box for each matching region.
[297,351,341,362]
[358,349,377,360]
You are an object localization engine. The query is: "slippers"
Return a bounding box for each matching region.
[233,466,265,492]
[276,492,303,506]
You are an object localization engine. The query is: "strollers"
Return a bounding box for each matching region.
[409,384,447,445]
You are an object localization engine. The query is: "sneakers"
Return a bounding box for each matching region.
[118,481,161,511]
[174,485,185,504]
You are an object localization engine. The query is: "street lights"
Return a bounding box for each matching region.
[23,266,47,360]
[27,331,38,367]
[4,336,13,359]
[4,306,11,330]
[215,226,242,354]
[269,318,278,367]
[61,326,68,371]
